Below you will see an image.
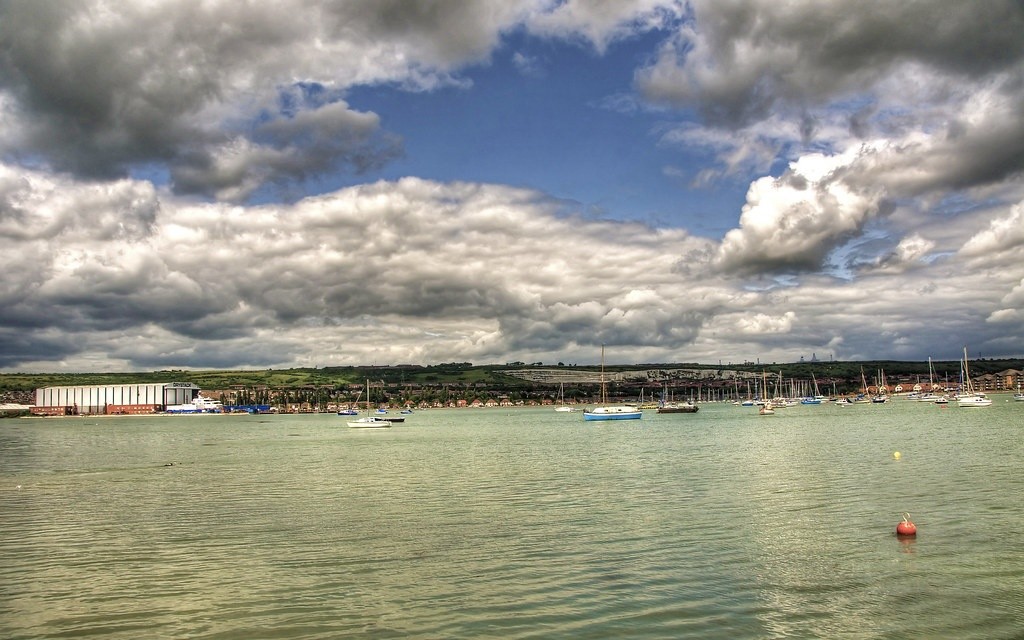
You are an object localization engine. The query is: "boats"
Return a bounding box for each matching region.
[1013,392,1024,401]
[906,390,950,404]
[655,398,700,414]
[374,406,389,415]
[401,404,415,414]
[367,416,405,422]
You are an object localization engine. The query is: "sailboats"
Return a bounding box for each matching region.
[337,400,358,415]
[346,379,393,428]
[732,366,888,414]
[636,388,659,410]
[553,382,575,412]
[583,344,642,421]
[953,347,992,407]
